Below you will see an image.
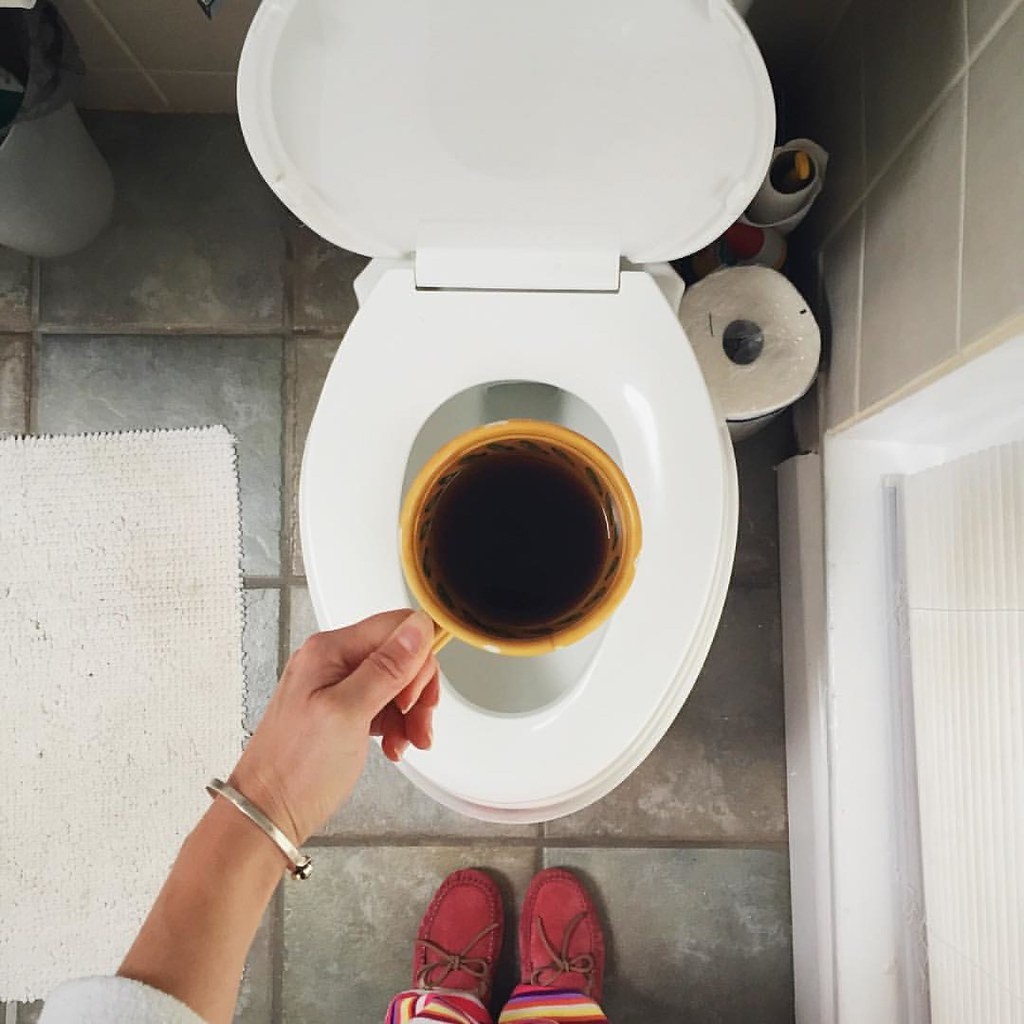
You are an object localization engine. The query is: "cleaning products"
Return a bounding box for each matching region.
[724,227,789,271]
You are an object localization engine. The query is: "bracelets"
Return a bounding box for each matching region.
[202,781,313,884]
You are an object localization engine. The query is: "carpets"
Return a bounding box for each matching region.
[0,423,250,1006]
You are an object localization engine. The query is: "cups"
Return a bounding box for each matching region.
[398,418,644,659]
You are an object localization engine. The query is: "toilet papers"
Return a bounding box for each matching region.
[673,257,831,437]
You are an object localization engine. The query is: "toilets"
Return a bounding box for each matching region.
[227,0,784,836]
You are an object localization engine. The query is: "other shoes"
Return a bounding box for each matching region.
[413,869,503,1012]
[519,868,604,1010]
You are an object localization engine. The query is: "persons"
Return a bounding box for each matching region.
[32,608,623,1023]
